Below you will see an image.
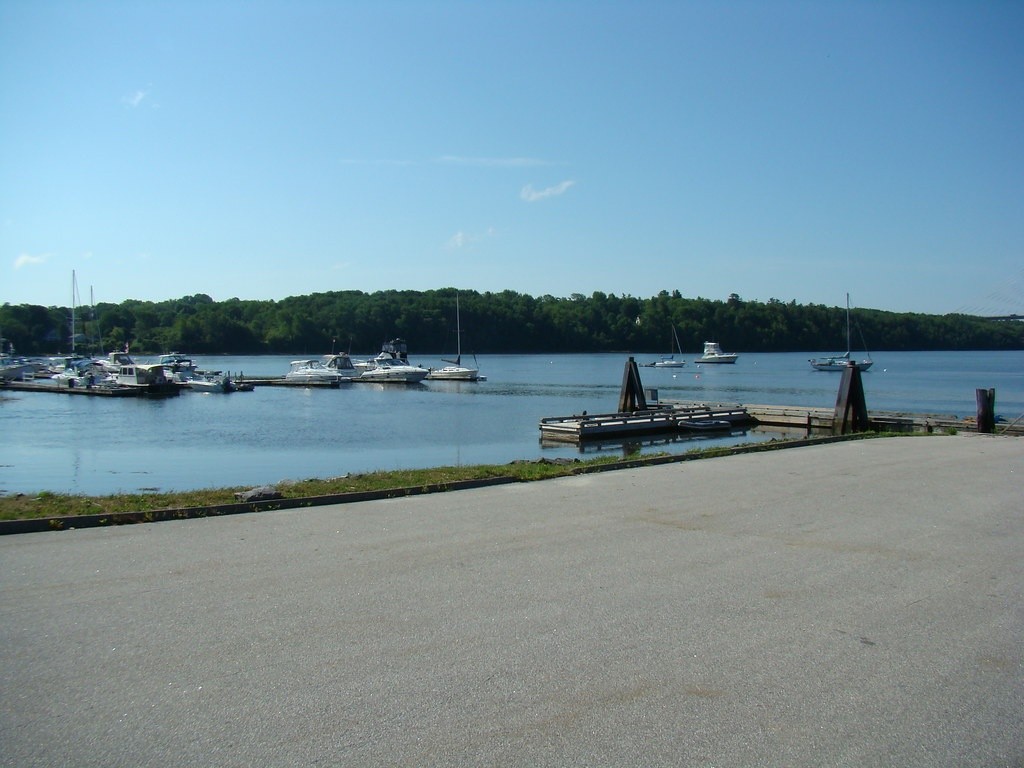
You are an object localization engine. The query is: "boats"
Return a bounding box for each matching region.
[677,418,732,433]
[694,340,740,364]
[285,291,488,381]
[0,270,256,396]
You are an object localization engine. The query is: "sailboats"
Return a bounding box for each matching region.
[809,292,874,371]
[650,321,686,368]
